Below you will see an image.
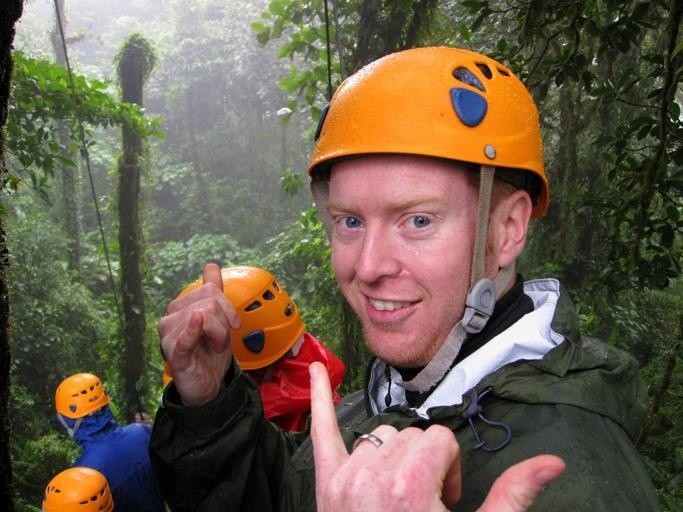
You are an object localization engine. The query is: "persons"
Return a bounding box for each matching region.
[158,264,348,436]
[145,42,670,512]
[53,372,168,512]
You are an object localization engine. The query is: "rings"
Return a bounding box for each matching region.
[351,432,383,453]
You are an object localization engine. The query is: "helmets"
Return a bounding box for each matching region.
[308,47,549,217]
[54,371,110,419]
[161,265,305,385]
